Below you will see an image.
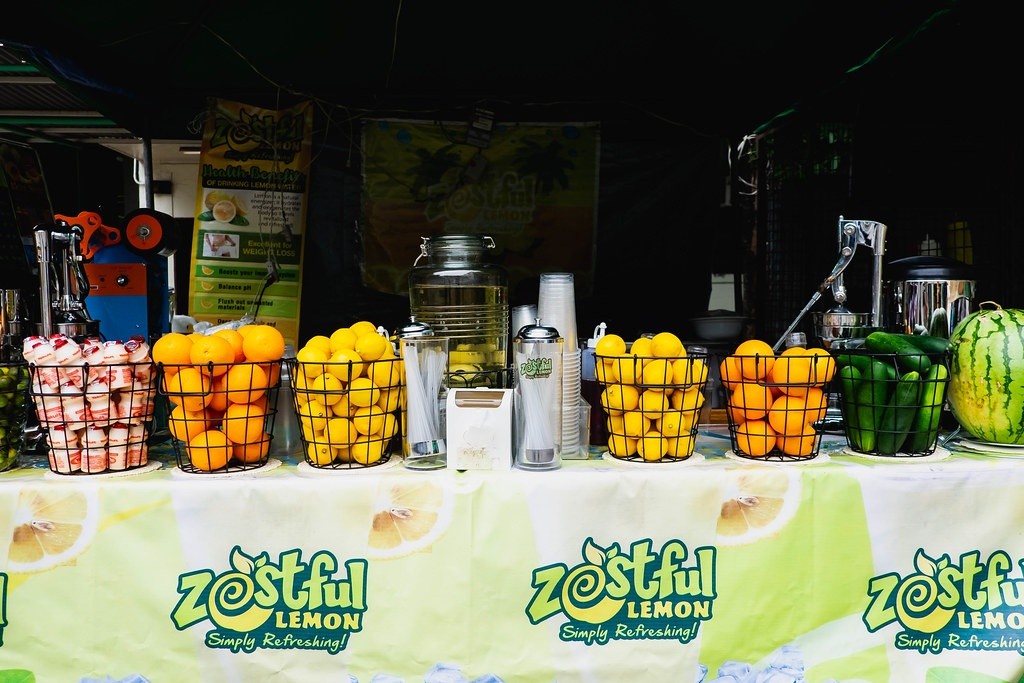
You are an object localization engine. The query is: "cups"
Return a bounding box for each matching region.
[512,272,582,472]
[400,336,452,469]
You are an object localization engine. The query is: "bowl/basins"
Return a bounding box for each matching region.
[693,315,744,341]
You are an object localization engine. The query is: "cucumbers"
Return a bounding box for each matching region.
[836,331,956,455]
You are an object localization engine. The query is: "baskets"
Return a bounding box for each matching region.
[834,348,953,457]
[591,351,712,463]
[27,362,155,475]
[154,358,284,474]
[283,357,408,470]
[715,352,840,462]
[0,363,30,471]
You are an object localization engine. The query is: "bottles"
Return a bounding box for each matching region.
[582,322,611,445]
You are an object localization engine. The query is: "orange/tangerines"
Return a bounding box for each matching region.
[153,324,285,471]
[720,340,836,456]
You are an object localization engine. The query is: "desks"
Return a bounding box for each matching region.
[0,422,1024,683]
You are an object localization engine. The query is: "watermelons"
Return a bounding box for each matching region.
[944,307,1024,446]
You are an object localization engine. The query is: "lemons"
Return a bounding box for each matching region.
[325,467,454,561]
[594,333,708,461]
[293,321,400,465]
[201,265,214,308]
[1,476,100,575]
[205,189,247,222]
[667,459,805,548]
